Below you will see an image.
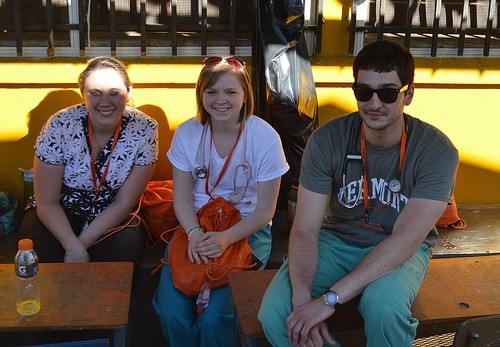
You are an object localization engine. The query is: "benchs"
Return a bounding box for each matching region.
[227,255,500,347]
[0,261,134,347]
[0,205,500,274]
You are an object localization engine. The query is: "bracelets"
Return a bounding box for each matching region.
[186,225,203,241]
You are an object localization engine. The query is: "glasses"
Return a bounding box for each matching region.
[352,80,409,104]
[201,56,247,70]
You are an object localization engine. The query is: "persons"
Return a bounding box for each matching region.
[257,41,458,347]
[31,56,158,347]
[151,55,291,347]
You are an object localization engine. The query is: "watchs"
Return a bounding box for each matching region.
[324,290,341,313]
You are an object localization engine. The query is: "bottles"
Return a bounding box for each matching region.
[287,178,299,232]
[15,239,41,316]
[19,167,34,210]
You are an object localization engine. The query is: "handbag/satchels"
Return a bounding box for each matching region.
[167,195,254,299]
[138,180,180,244]
